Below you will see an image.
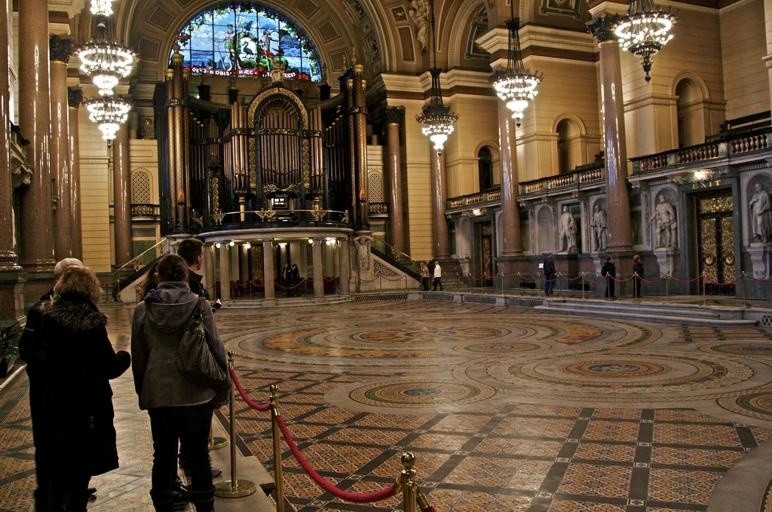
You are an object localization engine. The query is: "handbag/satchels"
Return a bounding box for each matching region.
[175,313,227,385]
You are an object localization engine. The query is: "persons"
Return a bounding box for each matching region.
[592,205,608,252]
[28,257,99,502]
[543,256,556,295]
[282,264,294,287]
[431,261,443,291]
[559,206,578,252]
[648,194,676,248]
[178,236,224,477]
[632,255,645,298]
[421,262,430,290]
[256,29,280,53]
[747,181,772,245]
[212,23,239,73]
[131,255,231,511]
[601,255,618,298]
[19,266,132,511]
[291,264,301,297]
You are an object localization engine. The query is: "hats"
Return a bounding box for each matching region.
[54,257,83,275]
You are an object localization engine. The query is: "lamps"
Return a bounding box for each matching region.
[73,0,142,150]
[609,0,680,83]
[415,0,463,156]
[488,0,544,128]
